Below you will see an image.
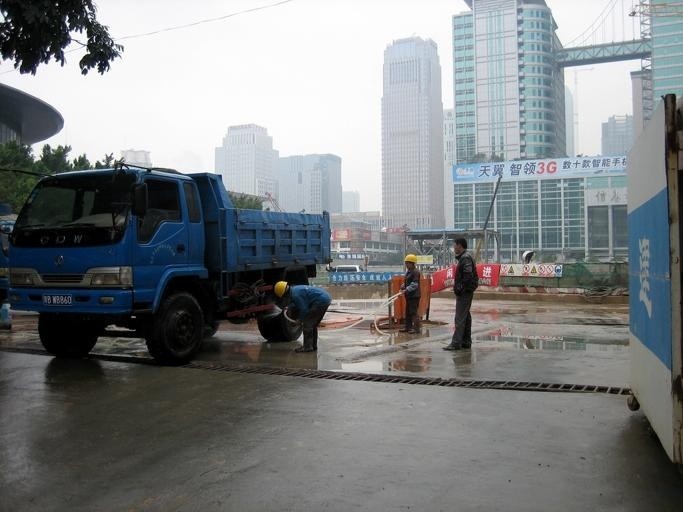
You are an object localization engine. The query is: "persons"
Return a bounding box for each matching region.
[398,254,421,334]
[274,281,332,353]
[326,266,336,272]
[441,237,479,351]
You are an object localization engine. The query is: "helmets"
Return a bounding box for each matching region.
[404,255,417,263]
[274,281,288,298]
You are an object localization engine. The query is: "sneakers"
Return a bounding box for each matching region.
[461,344,472,349]
[408,327,420,334]
[399,328,413,332]
[442,343,460,351]
[295,345,318,353]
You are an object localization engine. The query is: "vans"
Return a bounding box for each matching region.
[336,264,363,272]
